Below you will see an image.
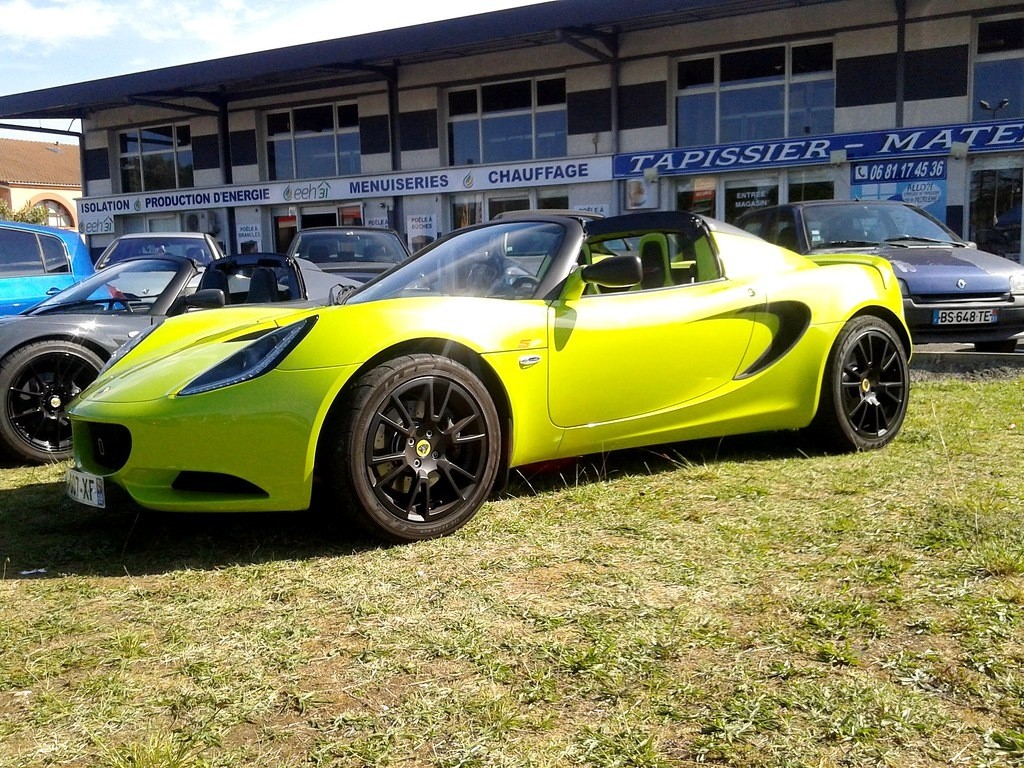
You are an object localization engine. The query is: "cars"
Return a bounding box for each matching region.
[287,225,411,285]
[0,220,130,317]
[733,199,1024,353]
[460,210,638,301]
[93,232,224,306]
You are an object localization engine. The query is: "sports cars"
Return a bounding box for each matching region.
[62,212,910,542]
[0,254,364,463]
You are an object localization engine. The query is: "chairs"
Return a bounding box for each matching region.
[201,270,232,305]
[776,227,813,254]
[243,269,280,304]
[308,246,330,263]
[186,247,205,262]
[627,234,675,290]
[363,245,390,261]
[576,244,601,295]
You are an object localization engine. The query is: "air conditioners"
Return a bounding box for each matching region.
[184,209,216,234]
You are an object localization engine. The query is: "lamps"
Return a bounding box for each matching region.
[830,149,847,167]
[47,119,75,152]
[980,98,1009,120]
[950,141,970,160]
[644,167,660,183]
